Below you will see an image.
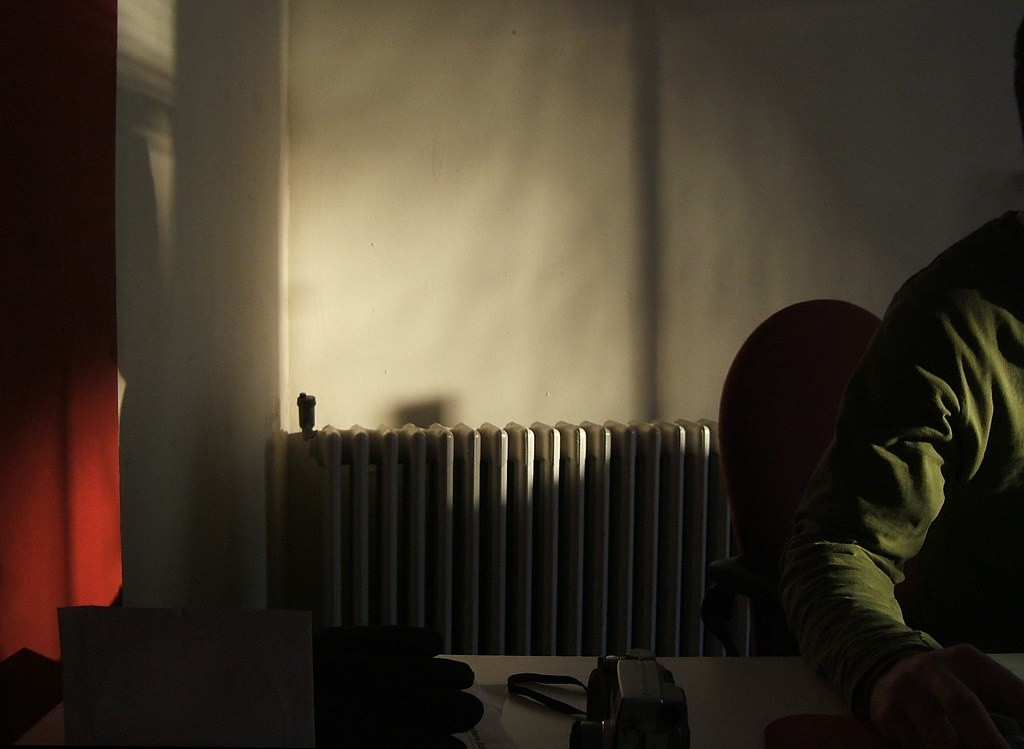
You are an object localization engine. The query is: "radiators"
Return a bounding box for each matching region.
[297,394,753,656]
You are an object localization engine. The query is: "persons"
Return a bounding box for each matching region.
[776,20,1023,747]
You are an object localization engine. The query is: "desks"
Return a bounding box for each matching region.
[10,650,1024,749]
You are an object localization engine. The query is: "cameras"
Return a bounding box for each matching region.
[569,651,690,749]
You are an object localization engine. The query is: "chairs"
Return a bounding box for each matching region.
[699,299,883,657]
